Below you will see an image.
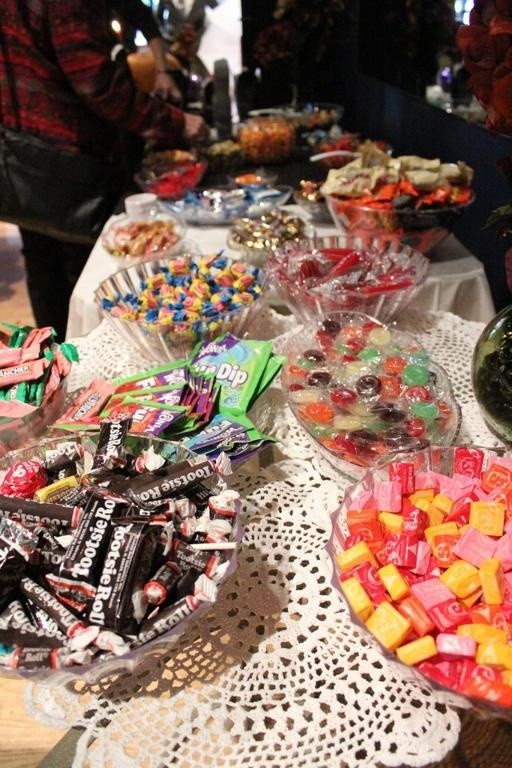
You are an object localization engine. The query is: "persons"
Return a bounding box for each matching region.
[1,2,212,355]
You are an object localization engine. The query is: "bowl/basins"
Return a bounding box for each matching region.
[2,320,69,451]
[319,182,478,264]
[284,103,345,149]
[92,252,268,369]
[265,233,431,326]
[196,137,244,176]
[133,160,209,201]
[225,228,320,269]
[295,192,332,224]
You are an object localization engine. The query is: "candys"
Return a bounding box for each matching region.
[0,325,79,423]
[0,423,275,670]
[75,334,286,420]
[144,151,203,181]
[300,179,321,204]
[169,183,281,212]
[303,126,356,142]
[288,319,453,444]
[322,144,474,209]
[234,211,306,247]
[113,218,181,254]
[269,242,415,293]
[337,447,512,705]
[102,250,263,322]
[208,139,240,153]
[302,103,338,125]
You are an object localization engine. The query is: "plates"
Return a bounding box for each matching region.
[324,443,512,723]
[0,430,242,688]
[469,301,512,451]
[62,383,280,475]
[278,310,463,471]
[174,184,294,226]
[310,139,395,172]
[100,212,188,264]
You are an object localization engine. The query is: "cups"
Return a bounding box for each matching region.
[123,193,159,217]
